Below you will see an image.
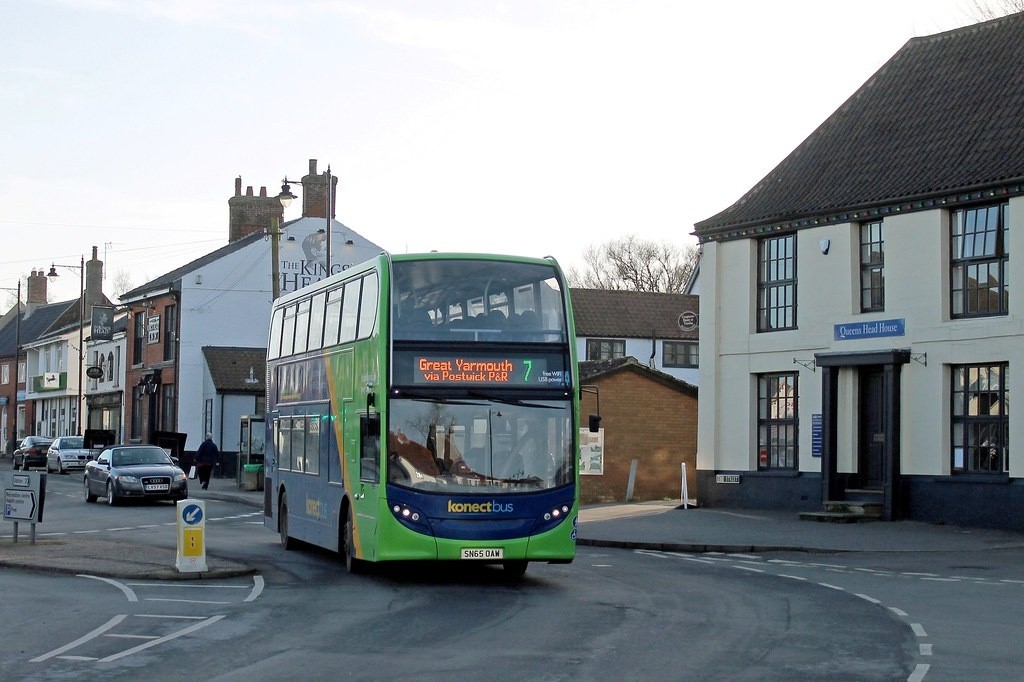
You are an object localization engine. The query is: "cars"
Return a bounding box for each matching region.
[12,435,55,470]
[83,444,190,508]
[47,436,102,475]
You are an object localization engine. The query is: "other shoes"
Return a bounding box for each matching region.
[199,481,208,490]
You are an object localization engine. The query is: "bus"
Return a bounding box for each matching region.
[263,251,602,573]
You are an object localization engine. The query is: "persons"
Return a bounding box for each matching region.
[389,423,410,460]
[194,433,220,489]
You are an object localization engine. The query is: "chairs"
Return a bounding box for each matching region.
[401,307,544,338]
[435,446,524,485]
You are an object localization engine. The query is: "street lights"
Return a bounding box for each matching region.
[136,373,158,442]
[278,165,332,276]
[47,254,85,432]
[0,278,22,452]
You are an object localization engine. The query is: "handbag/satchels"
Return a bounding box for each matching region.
[188,465,196,479]
